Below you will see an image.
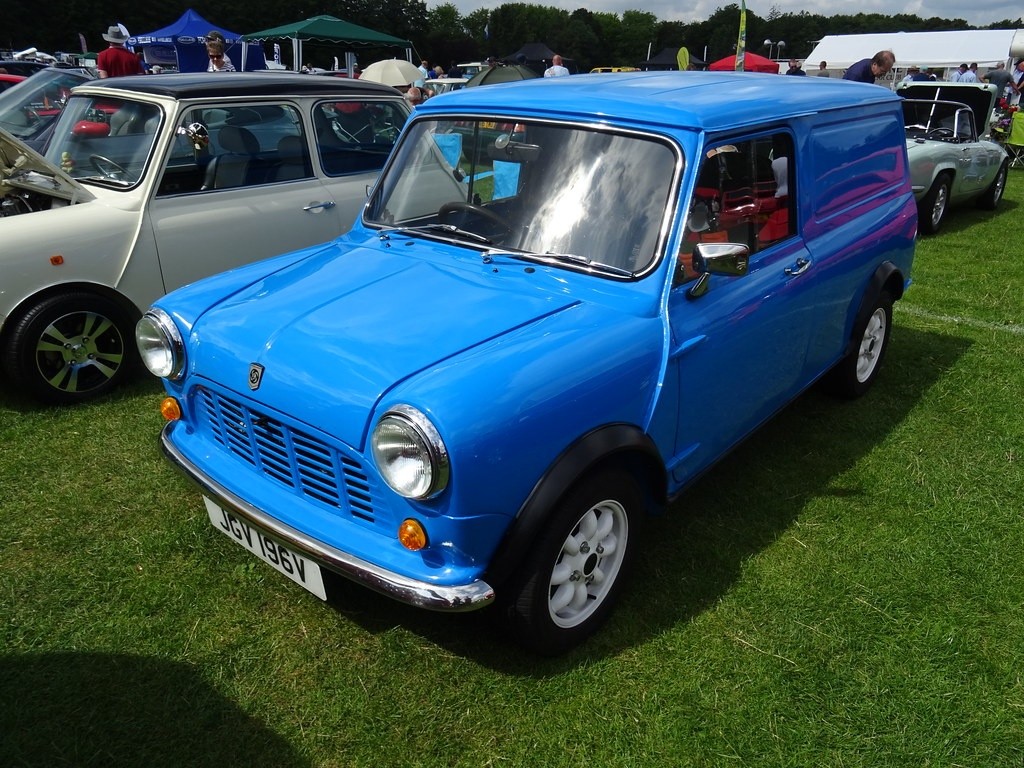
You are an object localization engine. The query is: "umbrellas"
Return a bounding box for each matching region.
[358,57,423,86]
[465,63,541,88]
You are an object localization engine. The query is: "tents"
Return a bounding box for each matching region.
[800,29,1024,93]
[125,10,266,73]
[640,48,709,71]
[498,41,579,76]
[707,52,779,73]
[241,15,413,80]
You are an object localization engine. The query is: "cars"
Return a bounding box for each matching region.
[450,118,524,152]
[0,65,299,199]
[135,70,915,653]
[673,146,918,280]
[0,72,468,414]
[893,80,1010,236]
[0,74,120,135]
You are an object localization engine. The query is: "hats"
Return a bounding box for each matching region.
[919,66,928,71]
[206,31,224,44]
[516,54,528,62]
[960,64,969,70]
[102,26,128,43]
[1014,58,1024,65]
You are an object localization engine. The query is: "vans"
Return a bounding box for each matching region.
[588,67,636,73]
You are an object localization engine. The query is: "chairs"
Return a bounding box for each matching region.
[200,125,308,191]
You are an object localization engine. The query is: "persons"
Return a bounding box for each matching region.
[842,51,895,84]
[353,63,362,74]
[687,63,697,71]
[414,61,463,96]
[136,52,162,74]
[949,58,1024,113]
[902,64,937,82]
[487,54,529,66]
[544,55,570,77]
[202,30,236,73]
[404,85,434,109]
[98,26,142,79]
[786,59,806,76]
[817,61,829,77]
[302,63,317,75]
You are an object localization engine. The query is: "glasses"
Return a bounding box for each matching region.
[208,52,222,59]
[790,65,797,68]
[819,65,822,66]
[879,65,887,74]
[206,38,216,42]
